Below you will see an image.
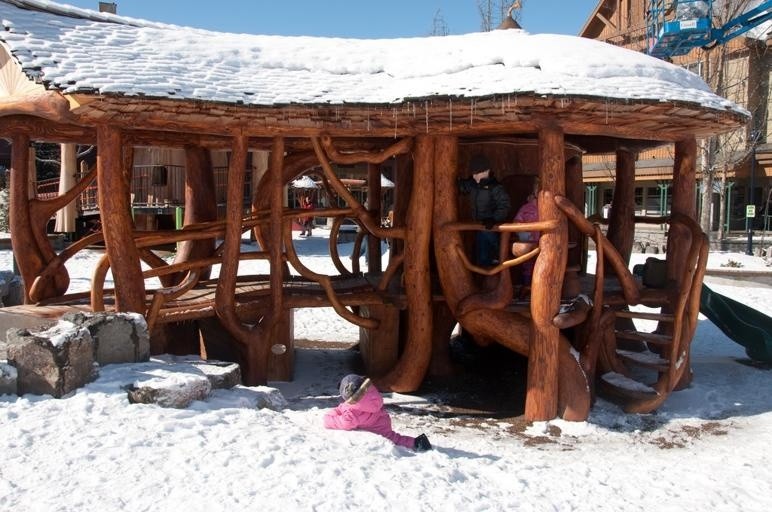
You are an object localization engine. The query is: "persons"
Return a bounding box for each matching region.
[453,155,513,291]
[385,210,394,248]
[349,224,368,266]
[322,372,433,451]
[300,196,315,237]
[514,175,543,303]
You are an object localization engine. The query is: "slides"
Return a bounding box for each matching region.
[633,258,772,368]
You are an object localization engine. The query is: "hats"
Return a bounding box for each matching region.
[470,156,489,174]
[339,374,364,401]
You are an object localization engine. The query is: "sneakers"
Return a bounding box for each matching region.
[416,434,431,450]
[300,233,312,236]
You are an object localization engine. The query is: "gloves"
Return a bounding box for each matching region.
[483,218,496,229]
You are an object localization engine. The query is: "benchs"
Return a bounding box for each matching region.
[0,267,697,420]
